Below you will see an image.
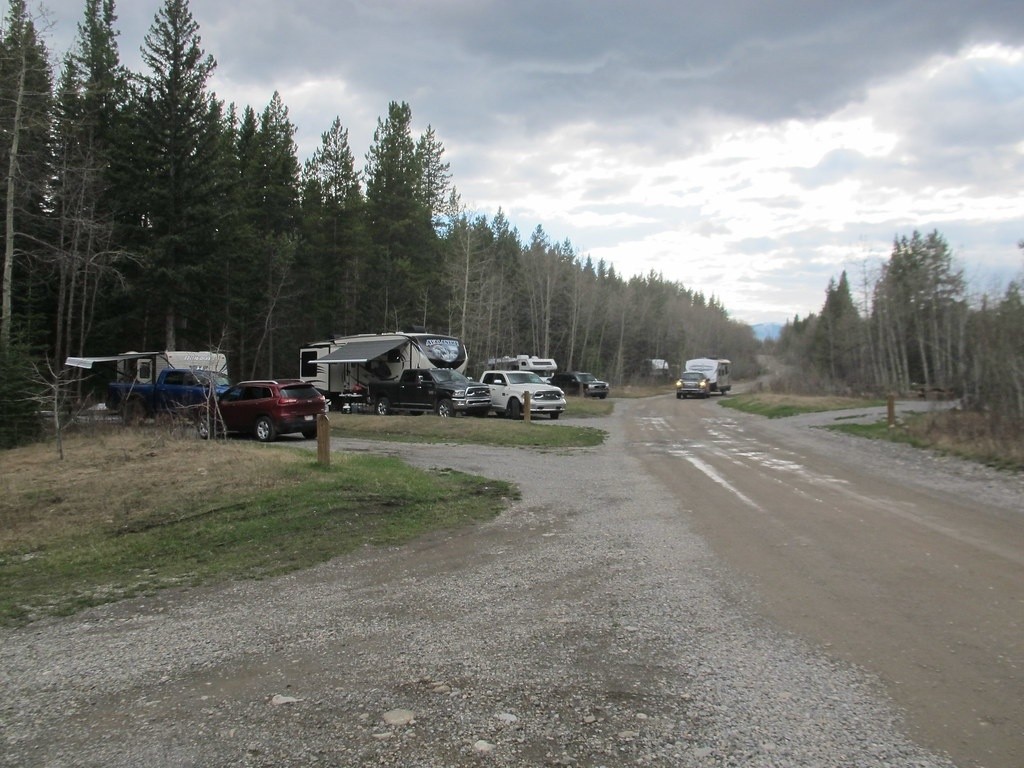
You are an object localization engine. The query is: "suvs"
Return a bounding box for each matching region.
[477,370,566,420]
[676,372,711,399]
[197,378,326,444]
[551,371,609,399]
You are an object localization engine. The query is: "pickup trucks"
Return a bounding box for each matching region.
[375,368,494,419]
[105,369,240,423]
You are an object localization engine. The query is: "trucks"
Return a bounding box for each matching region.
[488,355,558,384]
[685,358,732,394]
